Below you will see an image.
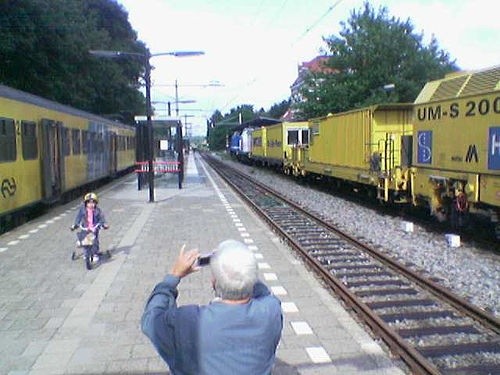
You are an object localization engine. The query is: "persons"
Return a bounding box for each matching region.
[71,193,108,263]
[140,238,284,375]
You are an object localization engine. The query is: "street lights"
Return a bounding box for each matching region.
[87,49,206,203]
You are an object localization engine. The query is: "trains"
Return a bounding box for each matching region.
[0,81,136,228]
[226,64,500,241]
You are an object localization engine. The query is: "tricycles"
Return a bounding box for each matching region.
[70,222,113,270]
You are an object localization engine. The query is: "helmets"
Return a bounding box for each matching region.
[84,192,99,201]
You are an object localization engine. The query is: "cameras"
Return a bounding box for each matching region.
[196,253,212,268]
